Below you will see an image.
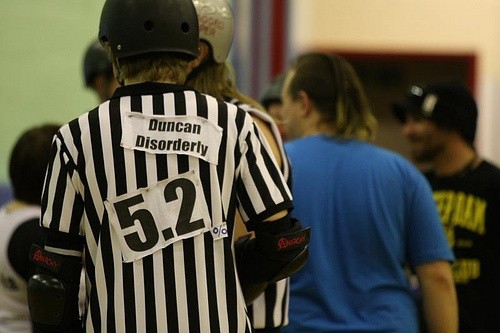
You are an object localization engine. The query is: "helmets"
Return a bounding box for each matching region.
[391,80,477,143]
[191,0,235,64]
[83,40,113,88]
[98,0,199,58]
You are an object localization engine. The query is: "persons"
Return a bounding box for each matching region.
[282,52,458,333]
[26,1,311,333]
[192,0,294,333]
[83,36,119,102]
[261,68,288,141]
[0,121,86,333]
[392,81,500,333]
